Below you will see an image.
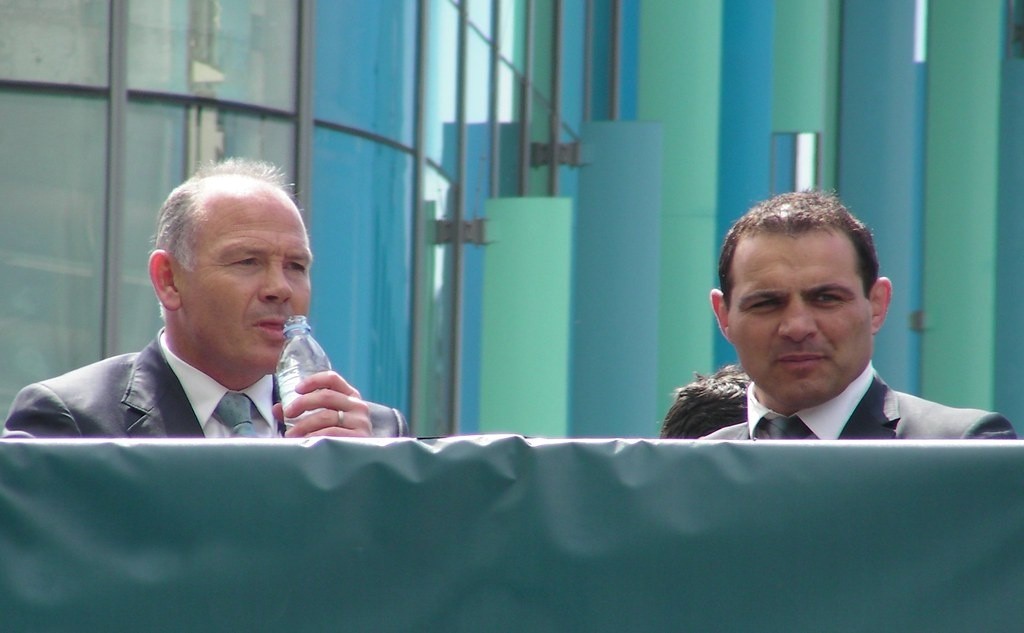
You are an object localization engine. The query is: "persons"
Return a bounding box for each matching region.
[698,190,1018,440]
[658,364,753,439]
[2,158,409,439]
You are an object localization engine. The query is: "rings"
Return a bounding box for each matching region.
[337,410,344,427]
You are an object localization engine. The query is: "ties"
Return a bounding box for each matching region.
[215,393,256,438]
[756,415,813,440]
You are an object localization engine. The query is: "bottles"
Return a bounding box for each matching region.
[277,317,335,437]
